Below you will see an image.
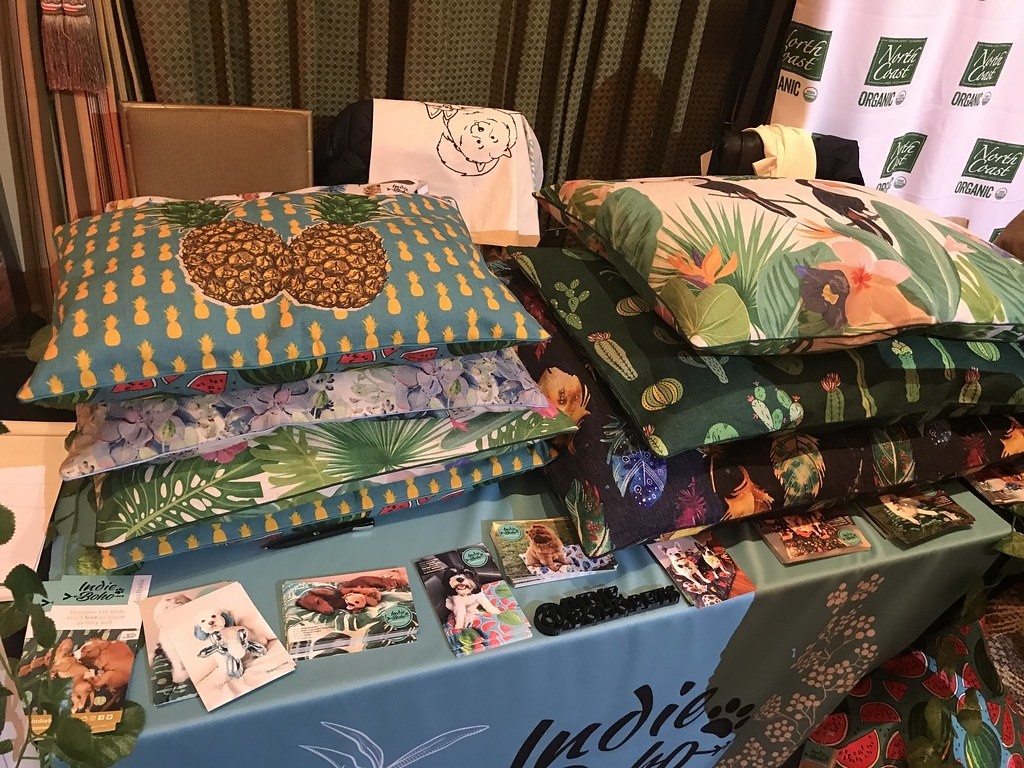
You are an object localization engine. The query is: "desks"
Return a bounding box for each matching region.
[0,421,1014,768]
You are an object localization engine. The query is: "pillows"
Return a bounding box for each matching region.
[516,295,1024,560]
[16,194,553,408]
[89,400,579,547]
[99,441,556,574]
[507,242,1024,458]
[58,346,546,482]
[103,179,428,212]
[531,177,1024,357]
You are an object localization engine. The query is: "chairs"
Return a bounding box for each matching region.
[332,101,545,187]
[699,130,866,188]
[118,100,313,202]
